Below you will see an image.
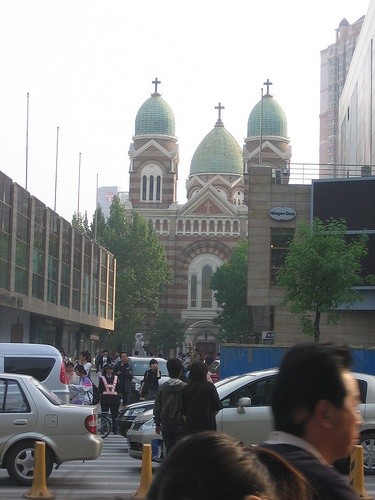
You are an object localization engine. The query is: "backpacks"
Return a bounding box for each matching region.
[83,377,100,405]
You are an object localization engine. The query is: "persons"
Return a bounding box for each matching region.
[60,345,224,453]
[256,342,369,500]
[146,430,313,500]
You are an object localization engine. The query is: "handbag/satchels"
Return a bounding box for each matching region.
[139,382,147,396]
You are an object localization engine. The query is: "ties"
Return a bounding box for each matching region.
[109,375,111,380]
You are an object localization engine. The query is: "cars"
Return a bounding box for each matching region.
[0,372,104,486]
[111,357,375,478]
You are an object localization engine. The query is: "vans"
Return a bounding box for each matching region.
[0,342,71,412]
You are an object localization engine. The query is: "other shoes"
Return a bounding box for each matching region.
[101,431,105,434]
[113,431,118,434]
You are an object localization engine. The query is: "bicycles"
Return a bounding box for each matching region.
[97,411,111,439]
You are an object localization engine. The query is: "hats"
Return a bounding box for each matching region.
[103,363,115,369]
